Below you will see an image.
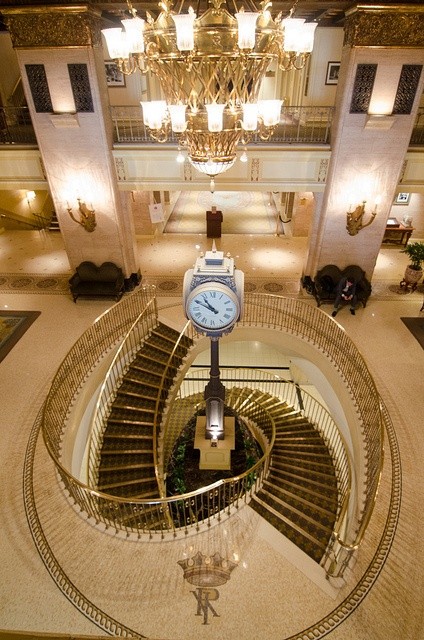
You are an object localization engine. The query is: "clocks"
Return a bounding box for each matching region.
[186,282,239,333]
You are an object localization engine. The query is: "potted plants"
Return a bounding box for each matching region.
[398,242,424,285]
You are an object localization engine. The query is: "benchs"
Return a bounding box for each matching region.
[68,261,124,303]
[311,265,372,308]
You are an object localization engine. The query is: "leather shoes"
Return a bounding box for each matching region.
[350,308,355,315]
[332,311,337,317]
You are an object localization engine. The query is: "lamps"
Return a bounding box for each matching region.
[26,191,36,208]
[346,176,381,236]
[101,1,318,196]
[64,171,97,231]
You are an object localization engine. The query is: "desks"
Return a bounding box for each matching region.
[382,220,415,248]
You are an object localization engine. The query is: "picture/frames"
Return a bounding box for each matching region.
[325,61,341,85]
[392,193,411,205]
[104,60,126,88]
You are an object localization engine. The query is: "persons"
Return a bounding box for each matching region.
[331,277,357,317]
